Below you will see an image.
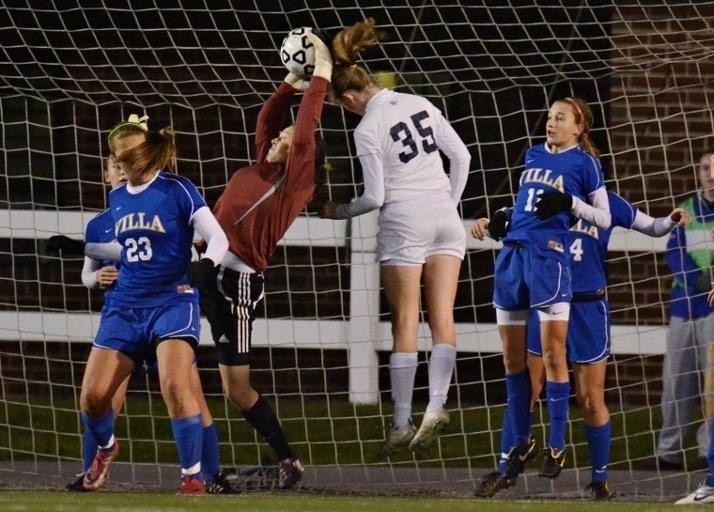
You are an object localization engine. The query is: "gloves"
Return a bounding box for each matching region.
[487,208,511,241]
[44,234,85,256]
[533,190,572,220]
[186,257,214,288]
[305,29,333,83]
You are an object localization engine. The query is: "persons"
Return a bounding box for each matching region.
[186,33,333,491]
[319,19,474,459]
[65,149,239,494]
[488,97,611,486]
[639,153,714,470]
[47,115,229,494]
[671,280,714,505]
[472,146,690,502]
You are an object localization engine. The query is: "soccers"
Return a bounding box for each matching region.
[280,28,335,77]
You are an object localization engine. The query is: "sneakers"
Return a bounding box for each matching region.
[408,410,450,453]
[64,473,94,492]
[177,477,208,497]
[206,473,248,496]
[473,469,517,498]
[501,437,536,479]
[270,457,305,492]
[673,484,713,505]
[383,421,417,457]
[82,438,120,489]
[536,447,566,479]
[584,481,610,500]
